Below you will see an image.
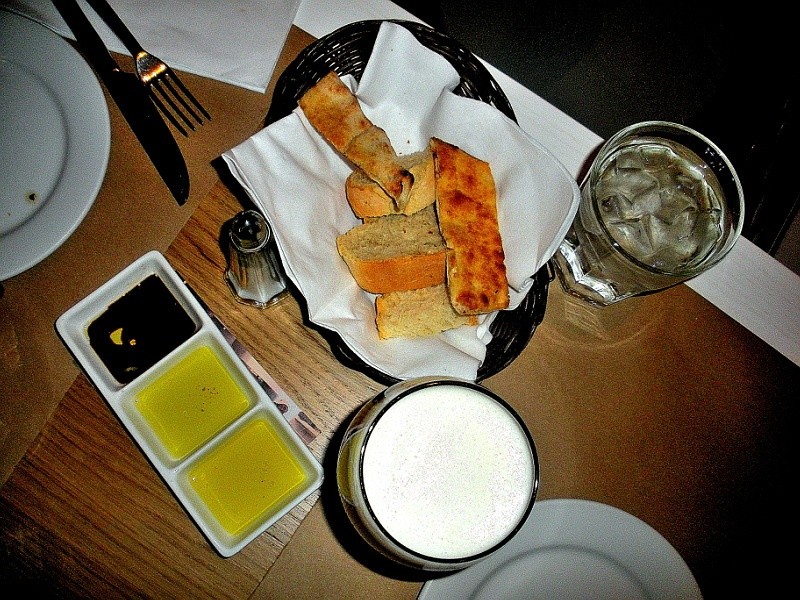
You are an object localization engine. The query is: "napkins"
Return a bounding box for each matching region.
[220,21,582,379]
[3,0,299,94]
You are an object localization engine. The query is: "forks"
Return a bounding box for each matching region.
[87,0,211,137]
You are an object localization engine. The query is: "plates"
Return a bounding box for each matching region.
[1,10,115,287]
[416,497,701,600]
[58,250,325,560]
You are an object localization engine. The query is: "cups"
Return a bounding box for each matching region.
[551,121,745,307]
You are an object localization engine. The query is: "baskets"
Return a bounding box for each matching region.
[263,18,549,387]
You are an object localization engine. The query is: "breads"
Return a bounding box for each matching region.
[301,70,513,338]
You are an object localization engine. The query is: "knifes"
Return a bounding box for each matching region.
[50,0,191,206]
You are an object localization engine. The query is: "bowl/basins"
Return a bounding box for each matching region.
[338,376,538,572]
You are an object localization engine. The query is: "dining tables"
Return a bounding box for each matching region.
[0,0,800,600]
